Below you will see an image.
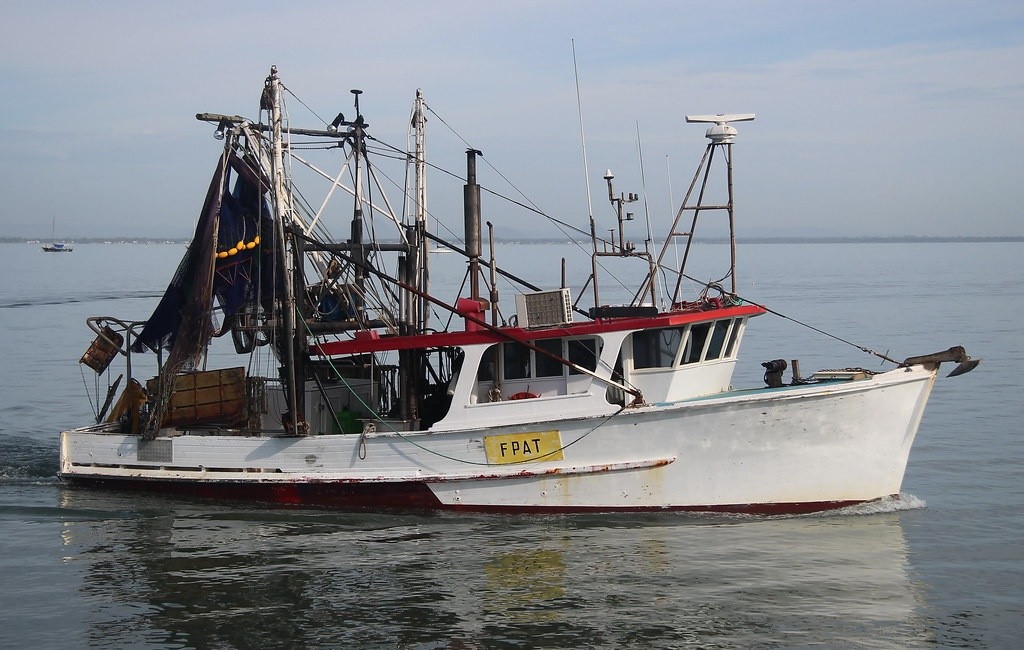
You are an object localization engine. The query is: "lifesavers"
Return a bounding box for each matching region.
[508,392,538,400]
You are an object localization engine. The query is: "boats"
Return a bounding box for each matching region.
[54,64,982,511]
[42,243,73,252]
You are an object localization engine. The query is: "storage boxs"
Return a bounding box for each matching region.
[330,410,365,435]
[360,418,422,433]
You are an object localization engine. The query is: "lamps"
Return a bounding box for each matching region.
[326,113,351,134]
[214,116,235,141]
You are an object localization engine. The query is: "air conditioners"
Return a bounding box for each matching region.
[515,288,574,330]
